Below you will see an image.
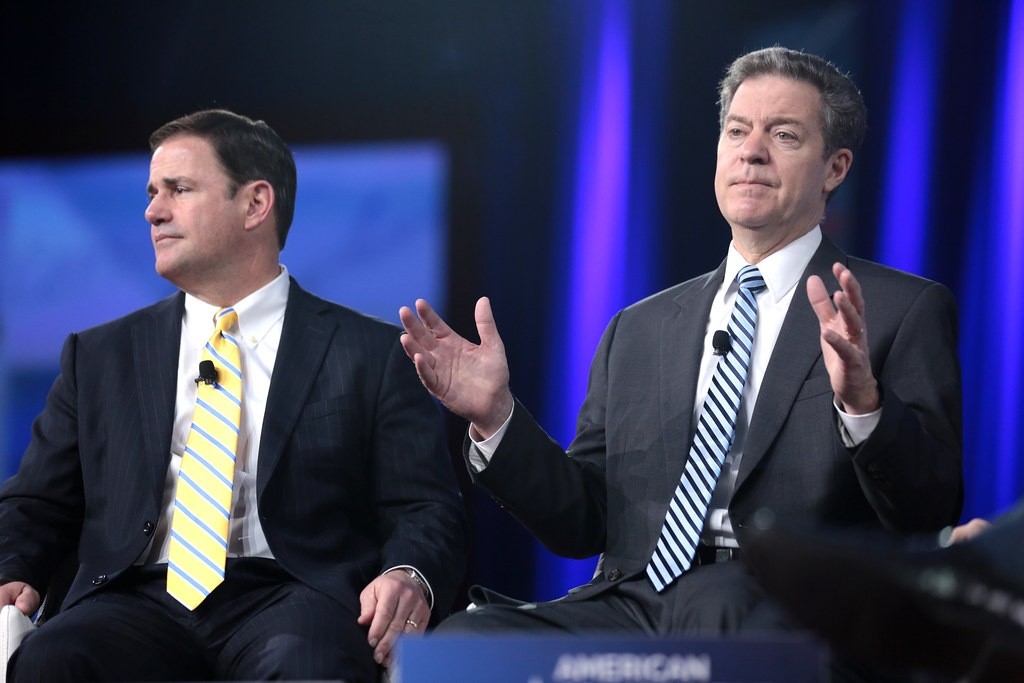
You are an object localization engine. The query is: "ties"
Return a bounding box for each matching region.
[166,307,243,612]
[646,265,767,594]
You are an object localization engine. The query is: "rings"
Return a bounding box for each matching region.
[844,325,863,340]
[407,620,416,627]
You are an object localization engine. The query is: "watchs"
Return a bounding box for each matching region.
[402,568,430,601]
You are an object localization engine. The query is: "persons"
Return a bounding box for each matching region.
[1,109,472,683]
[397,41,1024,683]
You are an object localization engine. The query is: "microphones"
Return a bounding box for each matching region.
[712,330,730,355]
[199,360,216,384]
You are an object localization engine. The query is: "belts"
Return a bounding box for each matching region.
[691,546,743,566]
